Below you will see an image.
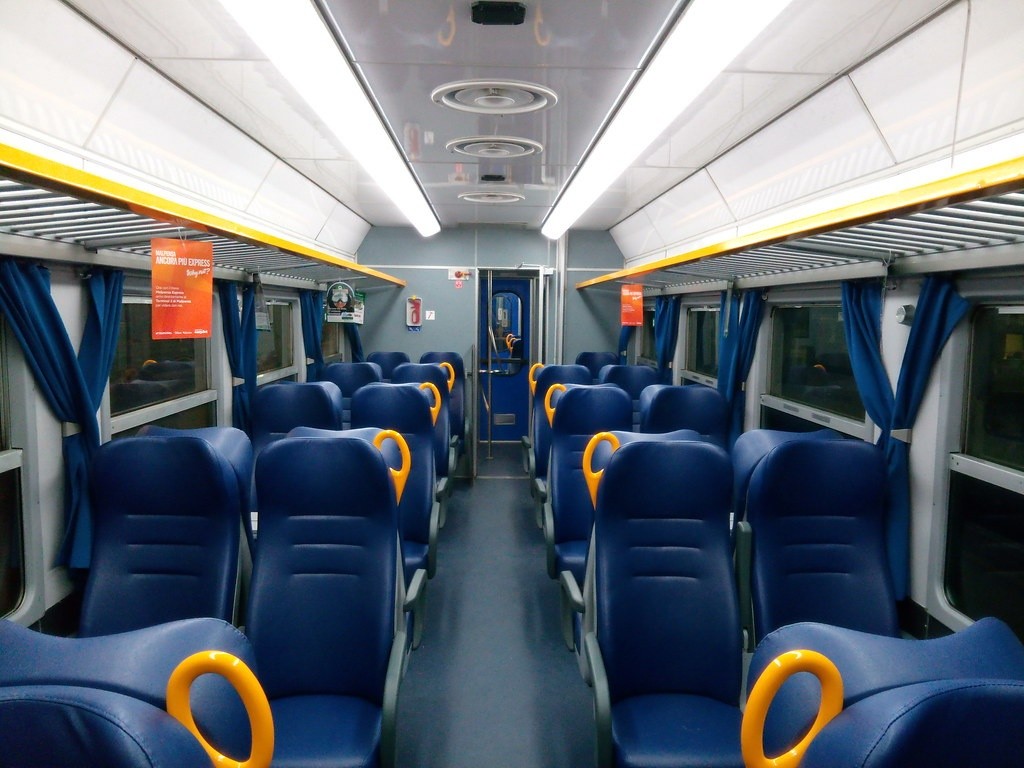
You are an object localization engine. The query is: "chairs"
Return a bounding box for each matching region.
[1,348,1023,767]
